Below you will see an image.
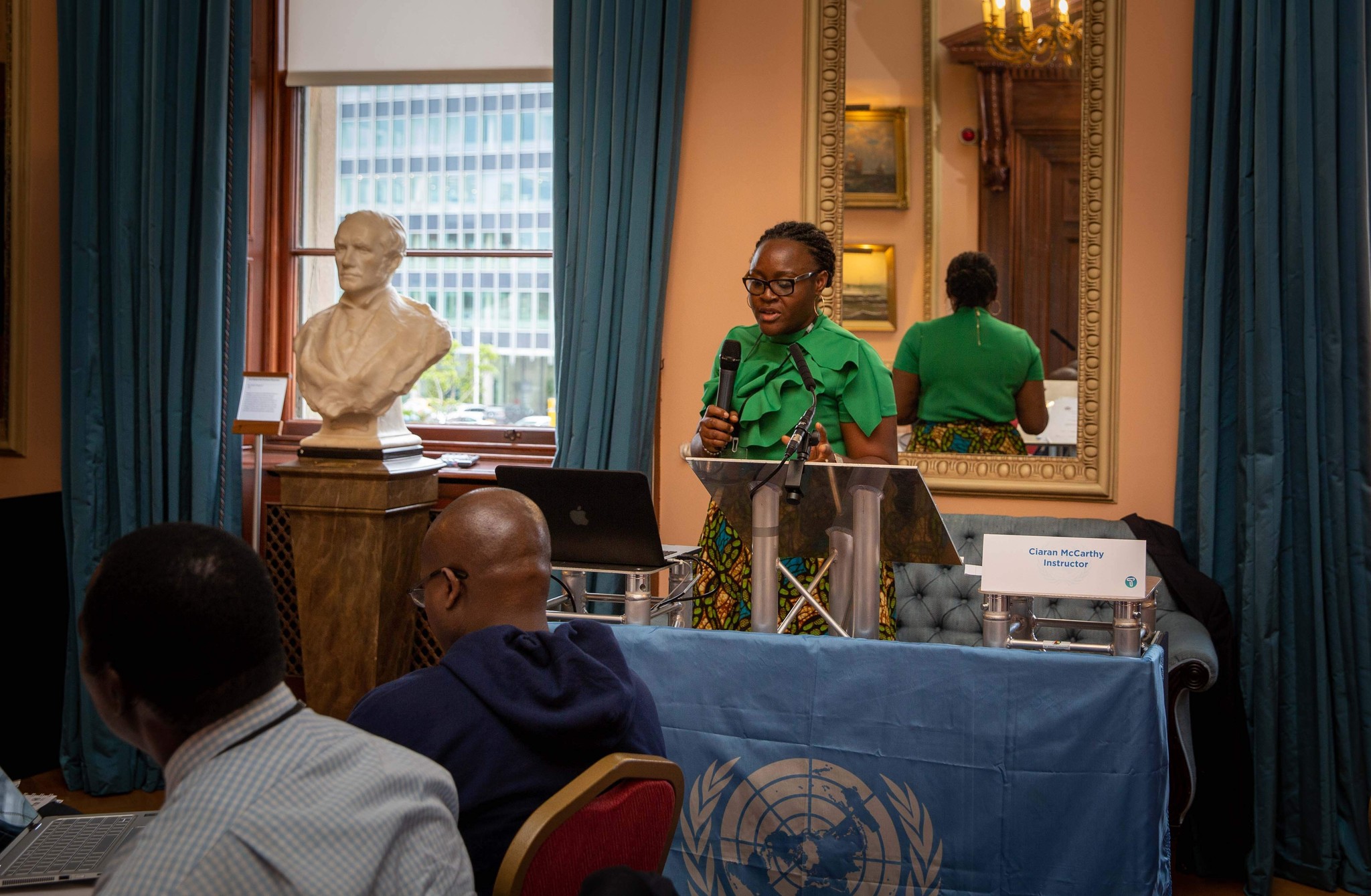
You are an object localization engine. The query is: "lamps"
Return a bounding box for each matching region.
[981,0,1086,70]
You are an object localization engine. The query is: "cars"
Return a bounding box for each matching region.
[438,404,552,427]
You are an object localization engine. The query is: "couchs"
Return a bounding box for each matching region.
[893,514,1219,828]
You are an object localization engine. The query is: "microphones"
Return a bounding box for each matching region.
[784,343,817,456]
[1047,329,1077,354]
[714,339,742,451]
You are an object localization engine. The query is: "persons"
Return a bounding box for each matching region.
[347,488,668,896]
[76,521,475,896]
[893,251,1049,454]
[292,211,452,448]
[691,221,899,642]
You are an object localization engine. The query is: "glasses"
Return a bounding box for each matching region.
[409,566,469,607]
[742,268,823,297]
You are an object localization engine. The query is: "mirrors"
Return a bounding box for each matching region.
[797,0,1125,502]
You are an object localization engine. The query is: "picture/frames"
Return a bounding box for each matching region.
[841,245,898,333]
[843,103,910,210]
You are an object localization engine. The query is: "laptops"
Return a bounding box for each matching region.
[495,465,702,567]
[0,768,160,896]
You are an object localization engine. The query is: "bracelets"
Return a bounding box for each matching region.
[702,445,726,455]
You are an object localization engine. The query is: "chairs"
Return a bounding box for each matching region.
[491,752,685,896]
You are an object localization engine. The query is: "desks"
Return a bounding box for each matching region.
[545,546,704,630]
